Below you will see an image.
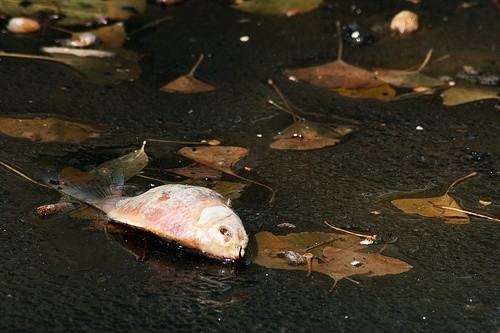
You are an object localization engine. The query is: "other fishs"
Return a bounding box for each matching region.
[39,168,249,260]
